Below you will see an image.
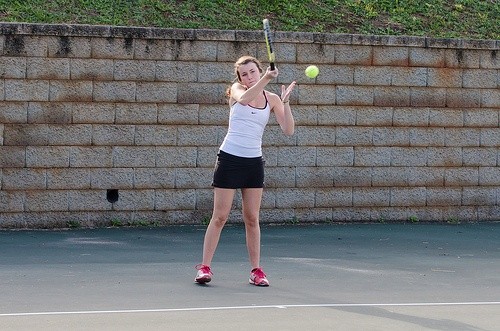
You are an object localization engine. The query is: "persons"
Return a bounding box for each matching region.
[195,54,296,287]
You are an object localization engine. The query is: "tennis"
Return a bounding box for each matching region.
[306,66,319,78]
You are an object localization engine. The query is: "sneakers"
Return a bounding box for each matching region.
[194,264,213,284]
[249,266,269,286]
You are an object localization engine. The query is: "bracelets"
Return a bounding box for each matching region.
[283,101,289,103]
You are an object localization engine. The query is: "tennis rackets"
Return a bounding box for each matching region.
[263,18,275,71]
